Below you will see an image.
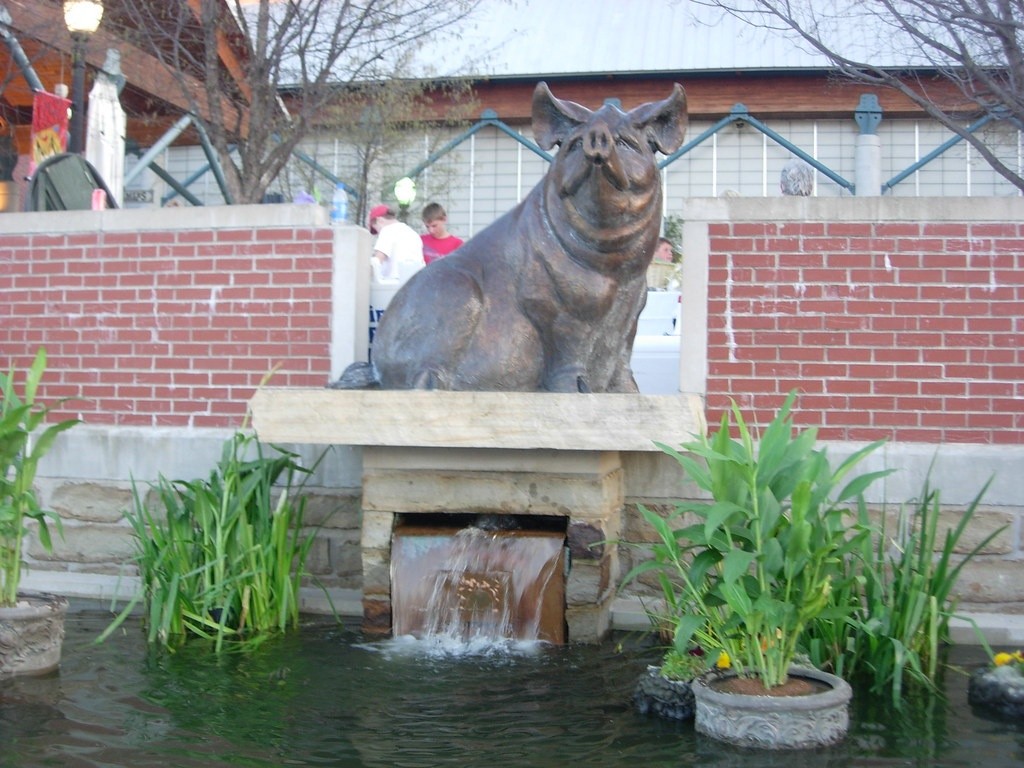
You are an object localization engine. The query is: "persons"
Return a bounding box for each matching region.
[369,206,426,282]
[653,238,674,262]
[419,203,464,265]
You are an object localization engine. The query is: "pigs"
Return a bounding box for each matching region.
[321,82,689,393]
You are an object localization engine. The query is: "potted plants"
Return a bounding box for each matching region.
[588,388,903,750]
[0,346,95,680]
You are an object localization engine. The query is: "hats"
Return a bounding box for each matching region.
[368,205,390,235]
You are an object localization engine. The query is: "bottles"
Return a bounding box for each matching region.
[330,183,349,226]
